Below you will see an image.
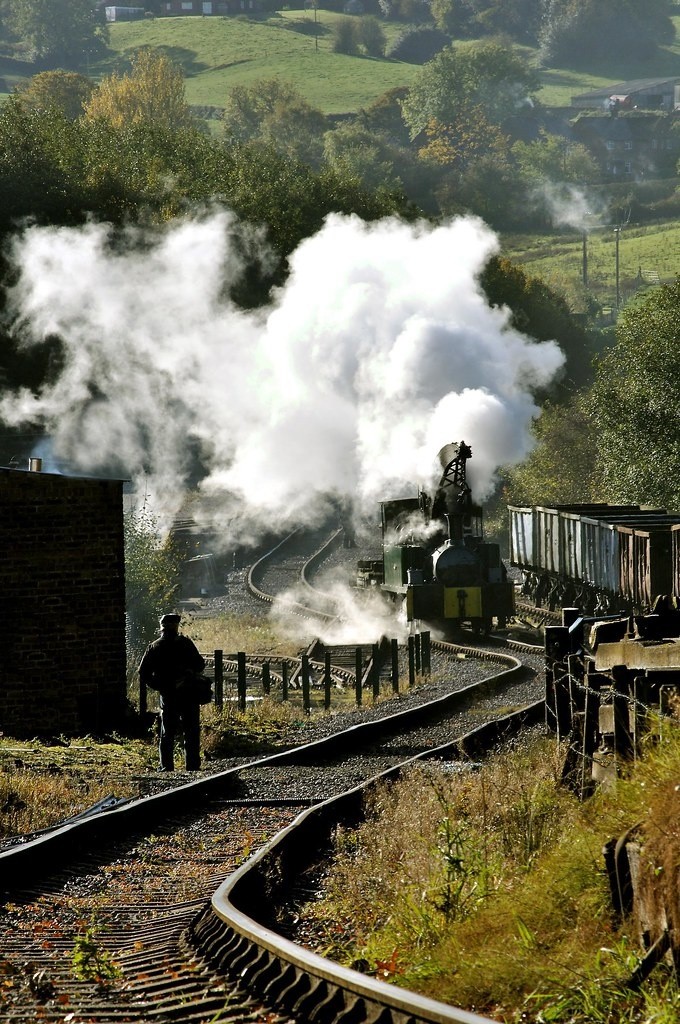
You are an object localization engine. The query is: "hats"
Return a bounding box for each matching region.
[160,615,181,627]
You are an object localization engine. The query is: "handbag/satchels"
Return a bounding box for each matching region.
[198,675,214,706]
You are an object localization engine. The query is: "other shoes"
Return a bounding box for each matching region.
[160,766,174,771]
[186,766,202,771]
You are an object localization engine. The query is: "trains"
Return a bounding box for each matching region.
[360,440,516,638]
[504,501,680,622]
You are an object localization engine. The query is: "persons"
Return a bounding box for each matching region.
[138,614,212,771]
[339,491,359,549]
